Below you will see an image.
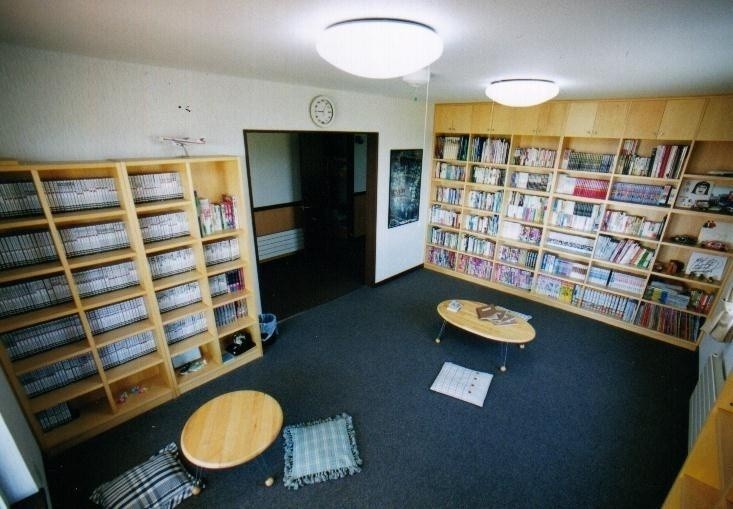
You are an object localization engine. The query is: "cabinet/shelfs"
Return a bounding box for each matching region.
[660,370,733,509]
[424,95,733,355]
[0,153,264,458]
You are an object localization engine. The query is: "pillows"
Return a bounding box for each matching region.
[88,443,204,509]
[430,362,493,407]
[280,411,364,488]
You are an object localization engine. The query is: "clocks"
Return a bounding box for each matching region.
[311,95,335,128]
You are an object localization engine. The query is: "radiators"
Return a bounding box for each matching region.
[688,353,724,455]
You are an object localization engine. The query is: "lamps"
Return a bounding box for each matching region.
[486,77,557,108]
[318,18,443,81]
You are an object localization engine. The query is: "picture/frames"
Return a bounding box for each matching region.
[388,148,423,228]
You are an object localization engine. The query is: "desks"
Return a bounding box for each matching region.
[180,390,283,497]
[435,298,536,373]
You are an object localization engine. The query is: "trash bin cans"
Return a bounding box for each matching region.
[259,314,276,341]
